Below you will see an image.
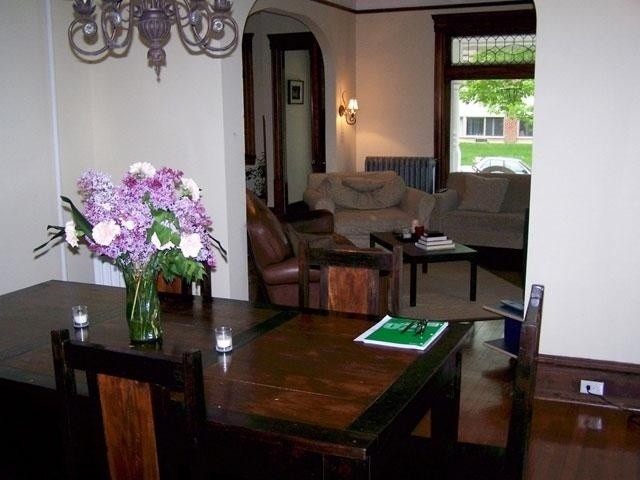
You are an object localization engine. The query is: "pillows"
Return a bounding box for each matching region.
[284,221,334,257]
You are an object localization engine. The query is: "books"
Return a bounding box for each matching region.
[364,317,449,350]
[414,230,456,251]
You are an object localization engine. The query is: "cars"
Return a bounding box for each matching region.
[460,155,531,173]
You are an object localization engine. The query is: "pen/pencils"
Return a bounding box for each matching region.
[401,320,415,333]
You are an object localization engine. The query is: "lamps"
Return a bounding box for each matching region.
[339,90,359,125]
[67,0,239,81]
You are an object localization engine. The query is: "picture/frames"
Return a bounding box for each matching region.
[288,78,305,105]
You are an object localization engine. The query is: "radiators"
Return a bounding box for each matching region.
[365,156,436,196]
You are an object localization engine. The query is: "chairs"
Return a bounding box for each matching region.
[303,170,531,259]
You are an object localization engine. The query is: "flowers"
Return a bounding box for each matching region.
[32,160,228,340]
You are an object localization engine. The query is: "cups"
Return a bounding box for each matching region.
[393,220,424,239]
[216,355,234,373]
[72,330,88,342]
[71,305,89,329]
[214,326,233,352]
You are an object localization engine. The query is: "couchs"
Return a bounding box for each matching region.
[245,188,389,314]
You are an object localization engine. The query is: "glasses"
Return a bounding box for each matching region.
[415,319,426,333]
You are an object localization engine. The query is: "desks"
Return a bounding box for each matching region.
[0,280,476,480]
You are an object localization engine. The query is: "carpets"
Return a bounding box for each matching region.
[388,257,525,322]
